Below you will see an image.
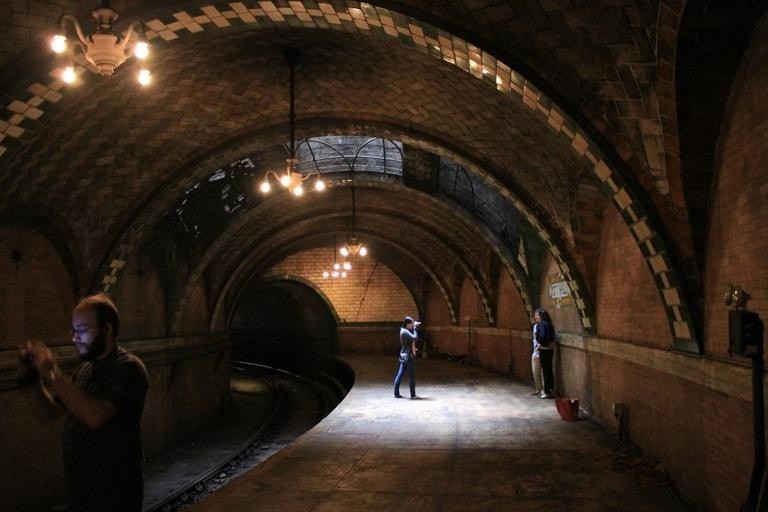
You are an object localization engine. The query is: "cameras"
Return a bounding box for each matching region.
[415,321,422,327]
[21,352,34,366]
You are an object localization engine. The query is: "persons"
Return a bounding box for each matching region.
[394,316,422,398]
[530,307,543,395]
[14,294,150,512]
[536,310,557,399]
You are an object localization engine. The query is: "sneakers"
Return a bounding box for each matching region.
[531,390,553,399]
[395,394,422,399]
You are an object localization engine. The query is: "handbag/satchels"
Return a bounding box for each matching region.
[399,352,408,363]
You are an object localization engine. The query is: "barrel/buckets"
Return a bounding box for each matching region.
[555,398,580,421]
